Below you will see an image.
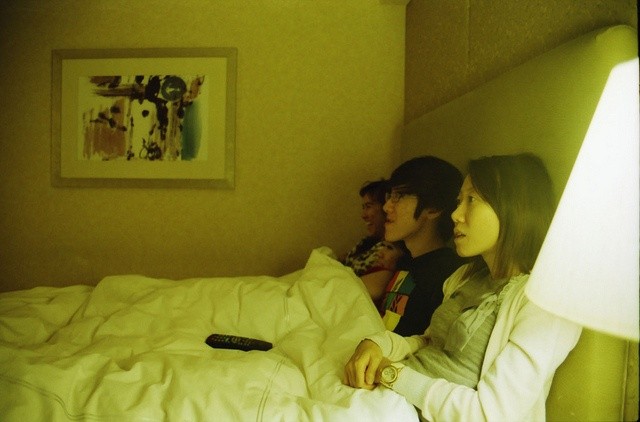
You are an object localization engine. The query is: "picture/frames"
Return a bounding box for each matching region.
[50,47,237,190]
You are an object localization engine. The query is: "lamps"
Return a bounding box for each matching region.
[524,56,639,342]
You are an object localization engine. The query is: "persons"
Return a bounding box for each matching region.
[372,155,491,337]
[342,152,583,422]
[336,179,405,310]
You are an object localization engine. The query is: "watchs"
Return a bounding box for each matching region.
[378,362,404,389]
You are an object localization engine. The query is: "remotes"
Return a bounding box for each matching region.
[205,332,271,351]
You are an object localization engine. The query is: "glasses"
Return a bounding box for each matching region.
[385,192,417,202]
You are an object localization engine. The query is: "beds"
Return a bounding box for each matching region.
[0,25,638,421]
